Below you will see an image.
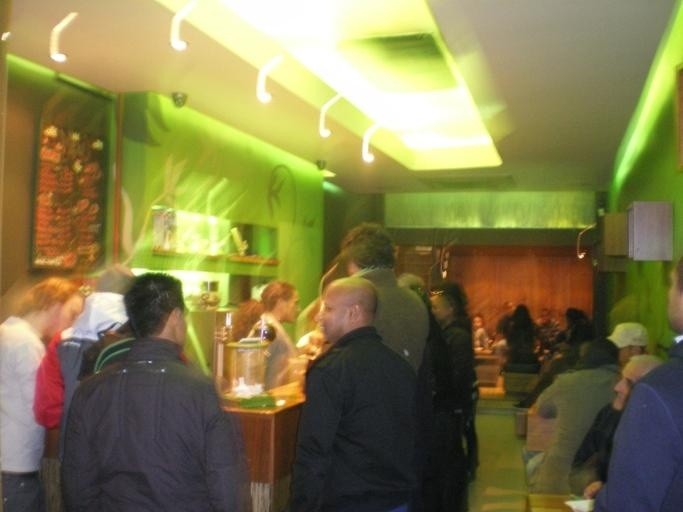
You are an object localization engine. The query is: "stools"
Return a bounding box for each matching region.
[503,370,539,394]
[474,354,501,387]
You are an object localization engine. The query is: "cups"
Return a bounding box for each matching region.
[225,338,269,398]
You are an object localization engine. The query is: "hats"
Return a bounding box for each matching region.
[606,321,650,351]
[621,353,665,382]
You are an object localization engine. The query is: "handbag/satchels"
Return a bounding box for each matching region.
[32,329,66,431]
